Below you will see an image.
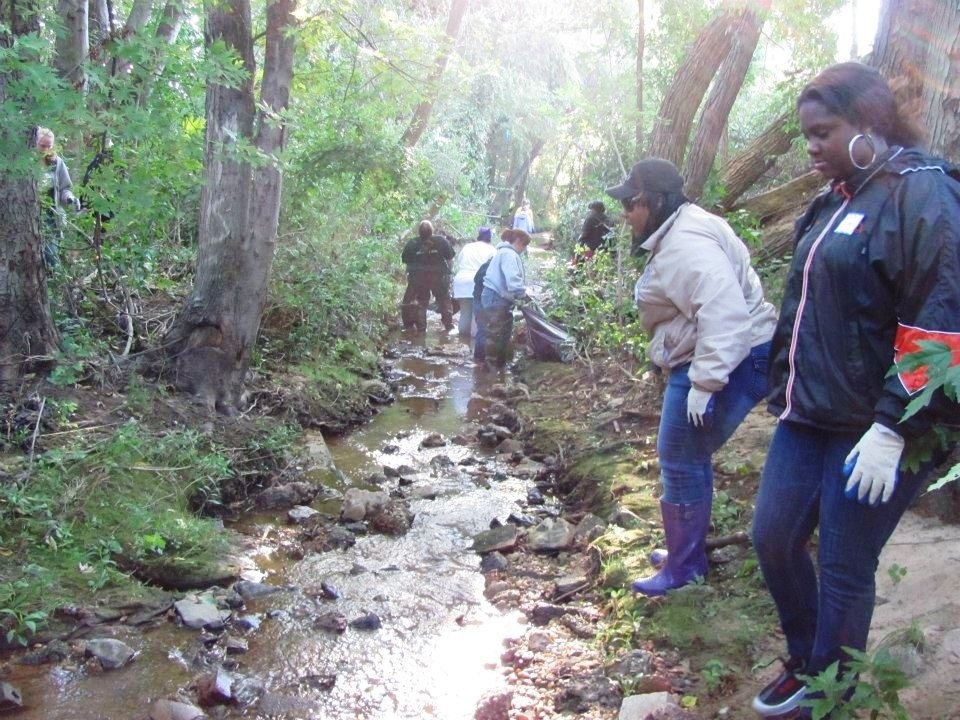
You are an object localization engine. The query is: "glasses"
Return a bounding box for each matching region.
[621,196,642,212]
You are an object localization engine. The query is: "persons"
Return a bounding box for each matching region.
[573,201,617,270]
[513,199,535,260]
[605,157,779,596]
[751,61,960,719]
[402,220,530,369]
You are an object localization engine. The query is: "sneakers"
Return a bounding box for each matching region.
[753,655,807,716]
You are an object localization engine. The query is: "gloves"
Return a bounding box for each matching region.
[687,387,715,433]
[842,422,906,508]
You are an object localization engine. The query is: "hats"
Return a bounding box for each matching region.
[605,158,683,200]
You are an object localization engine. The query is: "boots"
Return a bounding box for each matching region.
[632,494,708,595]
